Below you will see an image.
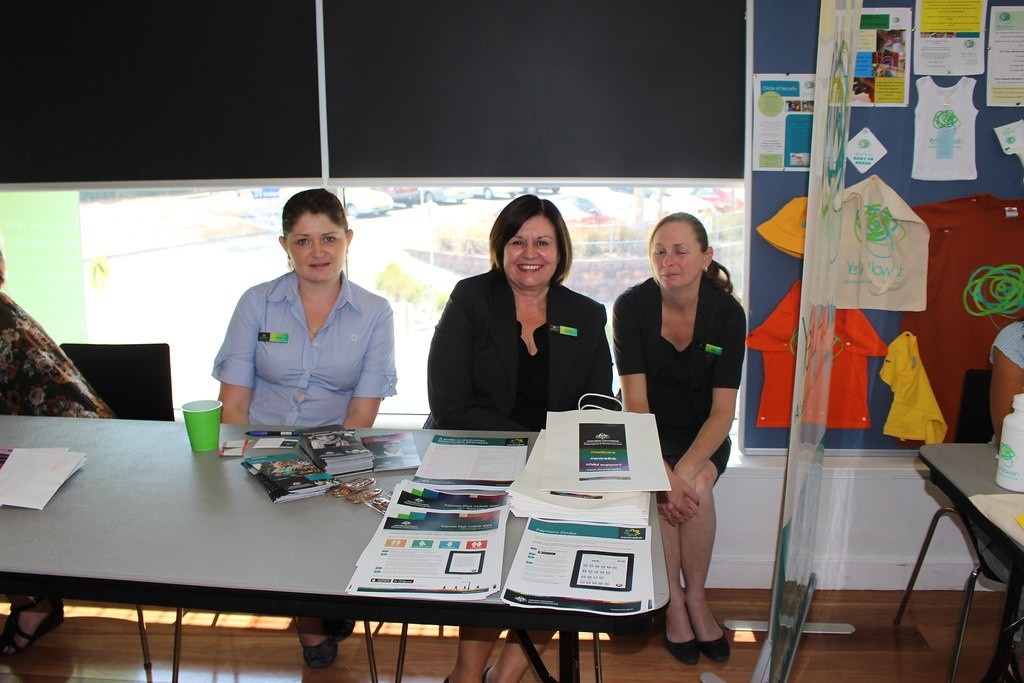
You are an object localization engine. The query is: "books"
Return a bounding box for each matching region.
[345,480,511,601]
[240,453,342,505]
[411,434,529,491]
[360,431,421,472]
[507,429,651,525]
[0,447,88,511]
[501,517,656,617]
[298,424,374,479]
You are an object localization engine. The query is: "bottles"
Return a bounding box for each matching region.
[996,393,1024,492]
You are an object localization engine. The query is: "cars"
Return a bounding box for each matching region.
[341,187,560,218]
[560,187,743,227]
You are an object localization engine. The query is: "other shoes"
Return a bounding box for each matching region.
[698,631,732,662]
[661,610,700,664]
[293,616,356,668]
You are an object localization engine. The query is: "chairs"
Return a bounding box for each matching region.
[58,344,175,670]
[893,369,1010,683]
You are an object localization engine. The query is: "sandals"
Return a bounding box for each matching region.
[0,590,65,661]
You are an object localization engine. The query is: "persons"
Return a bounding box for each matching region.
[977,317,1024,683]
[788,102,795,111]
[308,430,349,447]
[212,189,399,667]
[423,195,613,683]
[0,251,117,654]
[608,210,747,664]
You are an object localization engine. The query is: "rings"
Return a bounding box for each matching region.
[666,515,673,518]
[673,515,679,520]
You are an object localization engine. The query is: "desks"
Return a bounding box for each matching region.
[916,442,1024,683]
[0,413,671,683]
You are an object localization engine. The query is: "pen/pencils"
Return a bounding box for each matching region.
[245,431,298,437]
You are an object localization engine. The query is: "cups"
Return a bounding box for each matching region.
[181,400,223,453]
